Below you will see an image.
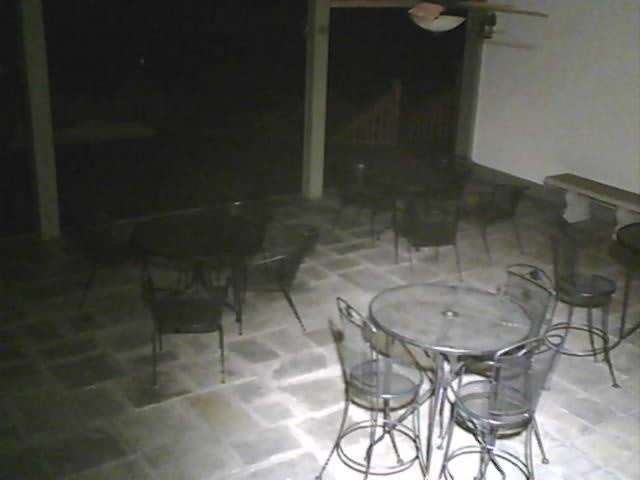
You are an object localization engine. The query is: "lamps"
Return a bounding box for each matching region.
[409,2,469,34]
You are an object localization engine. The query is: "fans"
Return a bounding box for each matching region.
[324,0,549,39]
